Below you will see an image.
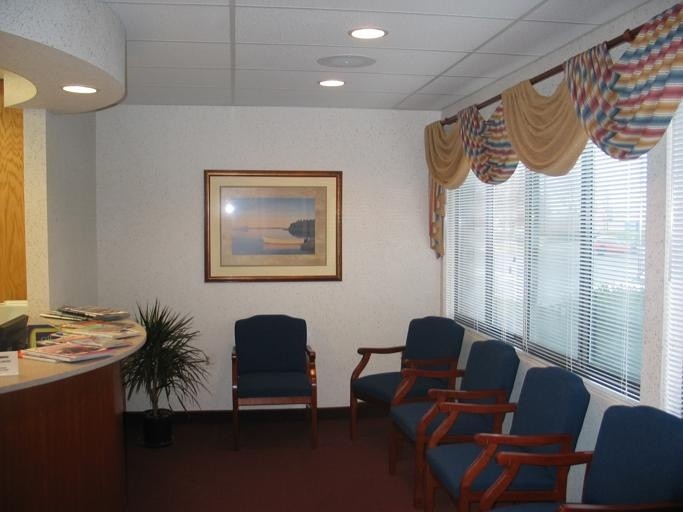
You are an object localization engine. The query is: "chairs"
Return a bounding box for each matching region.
[426,367,591,512]
[390,339,520,512]
[479,405,683,512]
[350,315,465,475]
[231,314,318,453]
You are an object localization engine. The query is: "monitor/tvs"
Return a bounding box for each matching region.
[0,314,29,352]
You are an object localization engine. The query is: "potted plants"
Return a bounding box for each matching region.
[121,299,214,449]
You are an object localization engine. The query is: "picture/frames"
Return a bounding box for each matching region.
[204,170,343,282]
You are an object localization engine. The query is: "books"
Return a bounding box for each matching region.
[18,305,141,366]
